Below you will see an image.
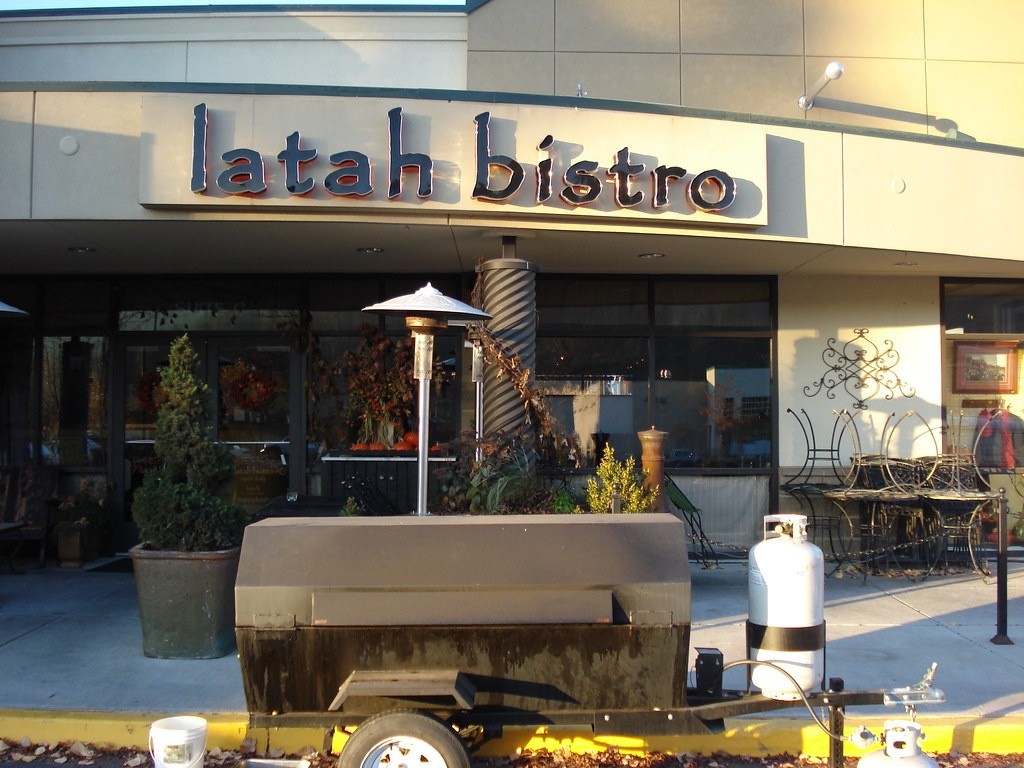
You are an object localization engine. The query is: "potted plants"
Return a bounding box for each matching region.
[51,475,119,568]
[129,333,246,659]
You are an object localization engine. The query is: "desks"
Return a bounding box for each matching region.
[781,482,865,575]
[917,490,1000,583]
[827,489,921,583]
[0,522,29,574]
[911,406,1000,499]
[783,408,862,491]
[834,408,915,500]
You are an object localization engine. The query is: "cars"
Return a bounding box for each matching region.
[261,435,339,461]
[669,448,706,466]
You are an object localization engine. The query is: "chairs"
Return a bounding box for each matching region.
[850,455,984,577]
[0,465,62,568]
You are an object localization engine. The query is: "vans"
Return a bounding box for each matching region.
[226,344,341,426]
[430,381,457,436]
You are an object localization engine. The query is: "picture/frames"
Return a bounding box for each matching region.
[952,340,1018,394]
